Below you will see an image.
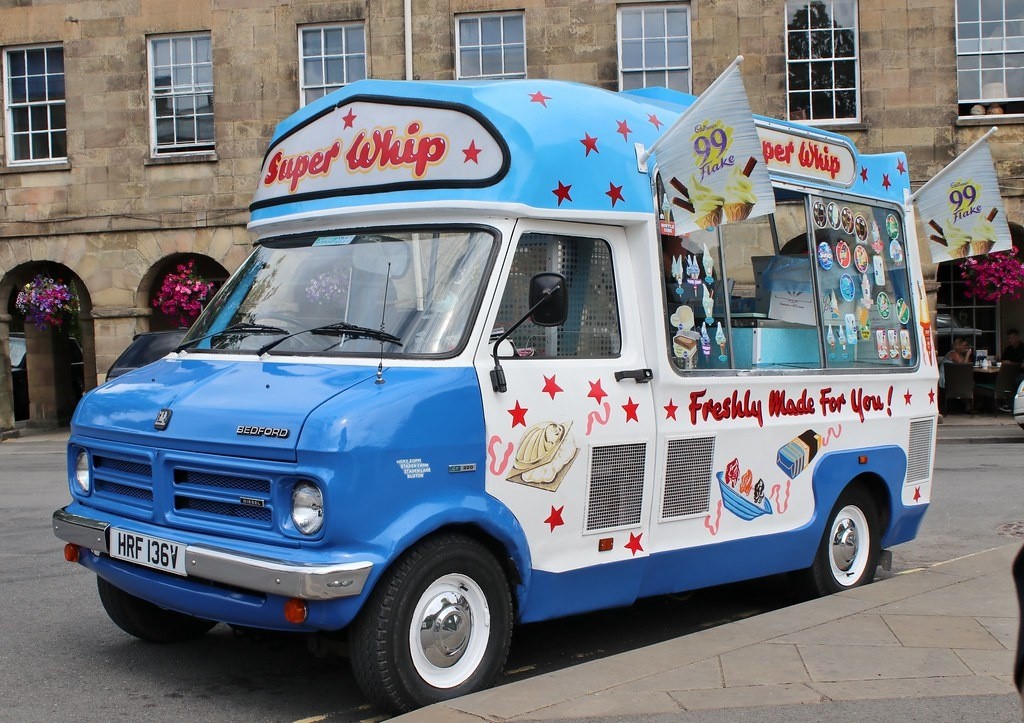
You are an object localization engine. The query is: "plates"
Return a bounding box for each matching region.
[973,366,981,367]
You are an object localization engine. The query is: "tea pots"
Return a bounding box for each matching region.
[980,359,989,369]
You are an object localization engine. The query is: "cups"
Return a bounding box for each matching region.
[996,362,1002,367]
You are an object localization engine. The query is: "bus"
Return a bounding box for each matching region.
[52,78,941,718]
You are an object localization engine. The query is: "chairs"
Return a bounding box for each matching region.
[944,362,975,418]
[976,363,1022,418]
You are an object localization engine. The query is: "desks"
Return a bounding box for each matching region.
[975,367,1001,373]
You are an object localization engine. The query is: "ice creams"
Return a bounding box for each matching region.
[827,324,847,354]
[670,155,758,230]
[672,243,726,357]
[928,207,1000,259]
[916,281,933,367]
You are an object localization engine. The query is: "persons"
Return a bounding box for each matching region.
[998,328,1024,412]
[939,338,978,414]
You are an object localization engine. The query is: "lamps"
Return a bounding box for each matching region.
[981,83,1008,115]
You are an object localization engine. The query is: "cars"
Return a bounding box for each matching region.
[105,330,189,383]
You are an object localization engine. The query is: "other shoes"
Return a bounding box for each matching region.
[998,403,1011,411]
[965,409,978,414]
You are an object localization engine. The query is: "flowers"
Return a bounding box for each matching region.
[15,272,80,337]
[153,257,220,328]
[959,246,1024,303]
[304,271,350,307]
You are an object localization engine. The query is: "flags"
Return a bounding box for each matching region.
[654,66,776,236]
[919,139,1013,263]
